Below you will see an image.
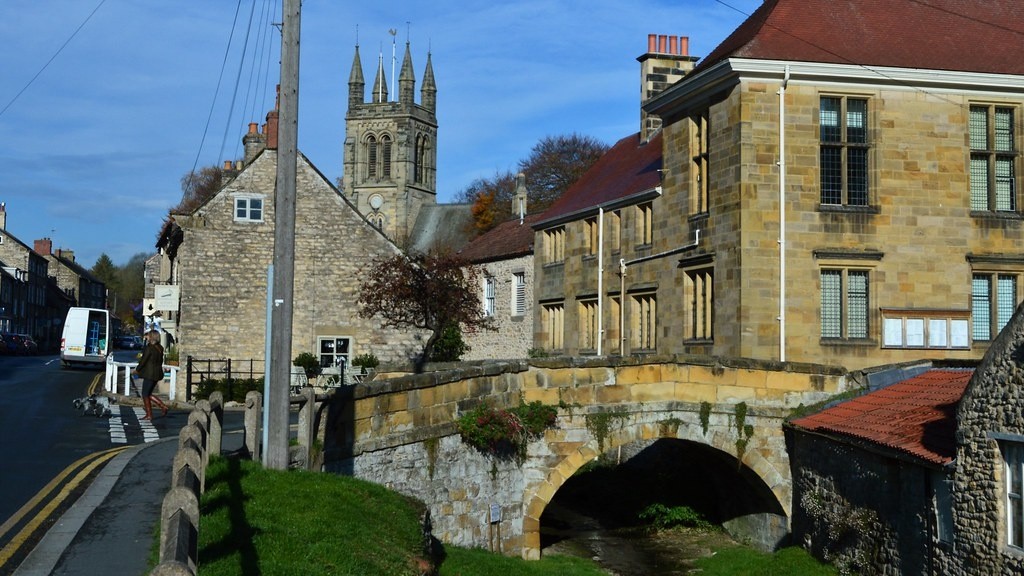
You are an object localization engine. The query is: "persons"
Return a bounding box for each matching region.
[133,330,169,421]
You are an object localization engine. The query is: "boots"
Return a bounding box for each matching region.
[139,397,153,421]
[150,394,170,416]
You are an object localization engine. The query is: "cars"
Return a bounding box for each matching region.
[3,333,39,356]
[119,335,143,350]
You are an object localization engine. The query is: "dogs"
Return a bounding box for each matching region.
[72,396,116,418]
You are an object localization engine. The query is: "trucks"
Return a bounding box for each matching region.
[59,305,114,373]
